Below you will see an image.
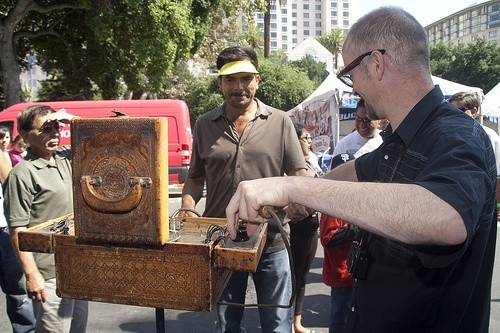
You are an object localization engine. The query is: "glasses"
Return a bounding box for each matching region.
[354,115,371,123]
[33,124,63,134]
[458,106,472,112]
[337,48,386,88]
[300,136,312,142]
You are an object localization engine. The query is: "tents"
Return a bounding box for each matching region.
[281,62,484,152]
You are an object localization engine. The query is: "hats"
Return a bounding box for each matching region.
[330,153,356,171]
[217,61,259,76]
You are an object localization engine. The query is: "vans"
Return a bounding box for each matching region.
[0,99,194,185]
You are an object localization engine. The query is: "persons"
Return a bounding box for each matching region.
[447,92,500,204]
[179,47,310,333]
[225,8,496,333]
[0,106,89,333]
[284,124,321,333]
[319,154,361,333]
